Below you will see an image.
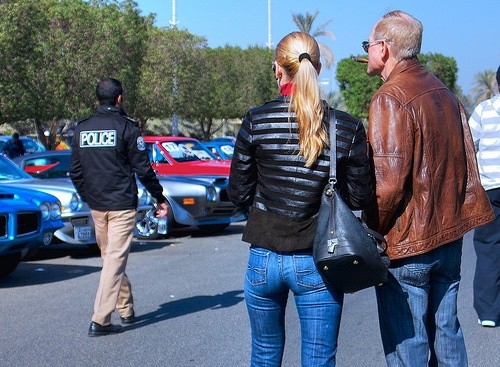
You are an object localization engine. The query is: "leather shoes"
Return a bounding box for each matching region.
[88,321,123,337]
[120,311,135,323]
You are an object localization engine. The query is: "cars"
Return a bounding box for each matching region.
[0,134,248,274]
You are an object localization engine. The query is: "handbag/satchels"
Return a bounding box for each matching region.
[312,108,390,293]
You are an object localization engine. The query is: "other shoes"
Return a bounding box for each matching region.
[478,317,496,327]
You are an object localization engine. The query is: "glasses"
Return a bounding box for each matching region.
[362,38,389,53]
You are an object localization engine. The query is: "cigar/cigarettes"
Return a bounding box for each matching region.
[353,56,369,63]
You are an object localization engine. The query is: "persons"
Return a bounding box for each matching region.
[68,78,169,337]
[365,11,500,367]
[3,132,25,159]
[225,31,375,367]
[468,65,500,331]
